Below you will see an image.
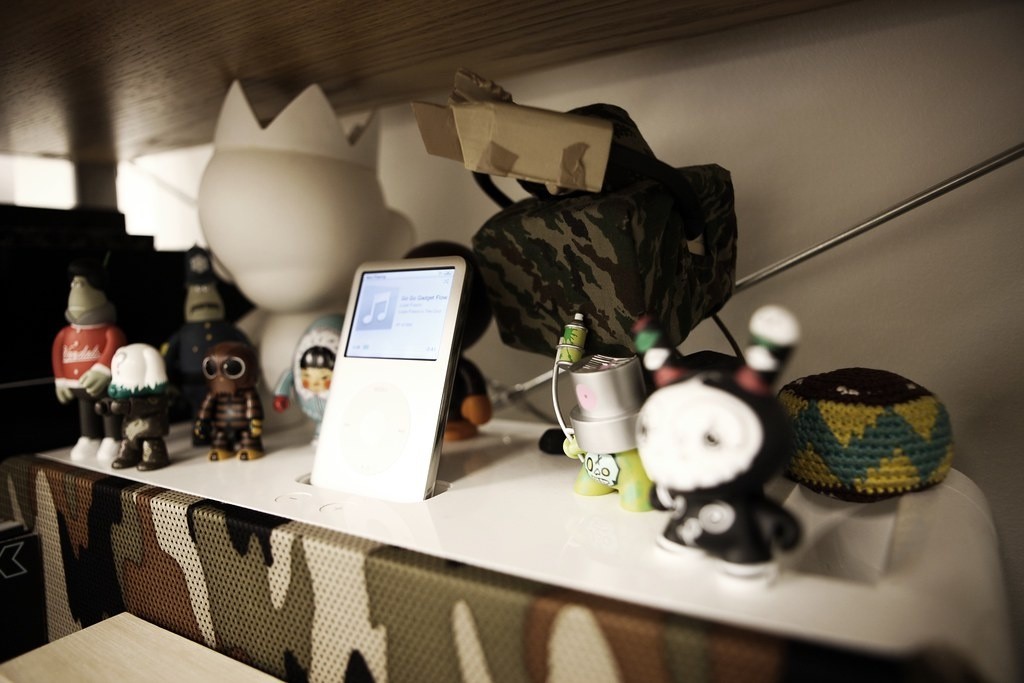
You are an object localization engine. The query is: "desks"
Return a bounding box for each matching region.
[0,416,1024,683]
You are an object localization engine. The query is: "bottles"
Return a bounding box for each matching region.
[557,313,588,368]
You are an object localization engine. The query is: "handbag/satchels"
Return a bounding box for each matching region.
[473,102,734,356]
